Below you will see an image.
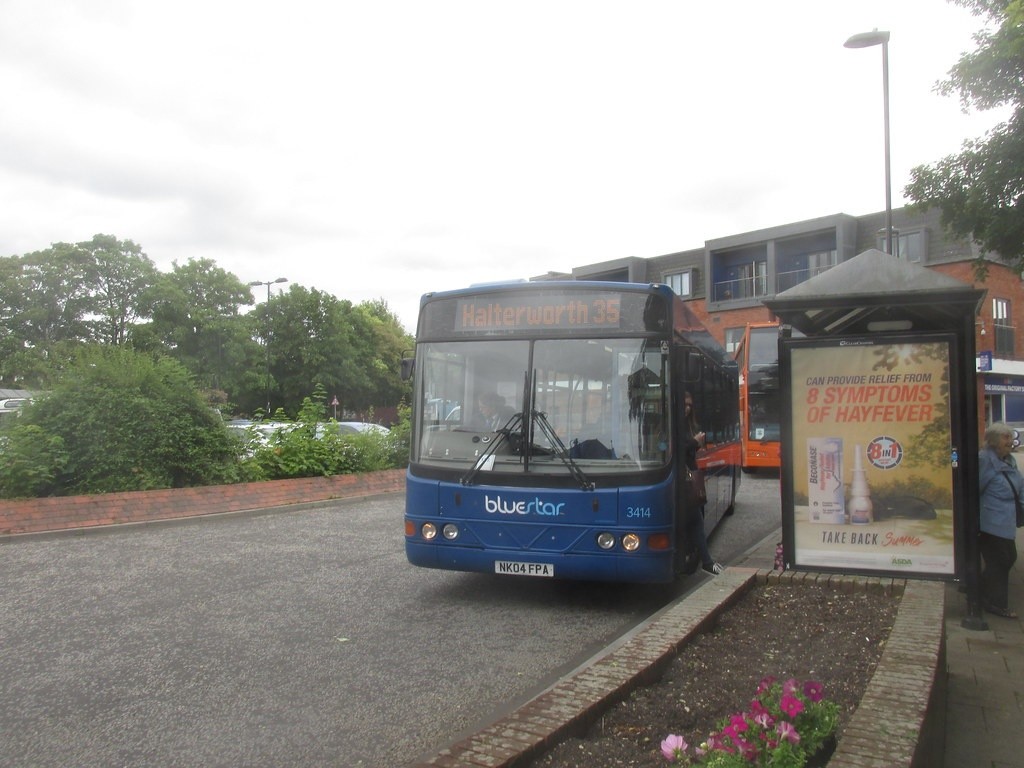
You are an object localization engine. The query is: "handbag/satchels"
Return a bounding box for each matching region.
[686,470,706,506]
[1015,501,1024,527]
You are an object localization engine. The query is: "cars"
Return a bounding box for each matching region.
[223,419,401,461]
[0,398,37,416]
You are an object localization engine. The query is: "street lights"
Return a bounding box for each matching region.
[844,30,895,257]
[248,277,287,421]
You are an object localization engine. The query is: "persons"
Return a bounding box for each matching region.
[684,389,724,577]
[977,422,1023,618]
[470,391,519,431]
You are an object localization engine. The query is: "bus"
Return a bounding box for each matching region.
[726,322,781,469]
[401,279,745,584]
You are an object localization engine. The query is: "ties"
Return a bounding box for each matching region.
[488,418,493,430]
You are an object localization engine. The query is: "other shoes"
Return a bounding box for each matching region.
[989,605,1018,618]
[701,562,724,577]
